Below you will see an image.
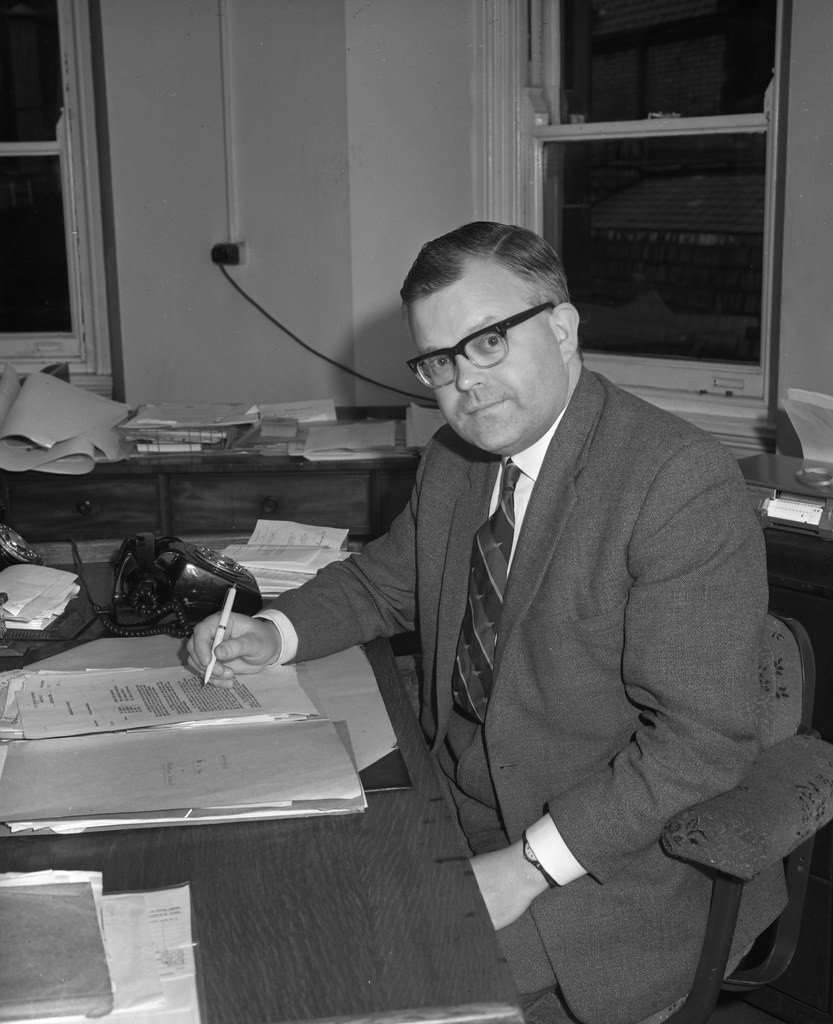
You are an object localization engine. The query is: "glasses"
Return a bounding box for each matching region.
[406,303,555,389]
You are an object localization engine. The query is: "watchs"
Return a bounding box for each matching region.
[522,831,557,889]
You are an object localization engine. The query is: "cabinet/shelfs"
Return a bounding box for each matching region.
[0,404,437,543]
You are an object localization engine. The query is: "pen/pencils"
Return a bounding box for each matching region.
[201,581,239,690]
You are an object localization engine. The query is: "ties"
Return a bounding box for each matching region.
[452,458,521,723]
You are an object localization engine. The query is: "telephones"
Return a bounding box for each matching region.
[112,533,263,618]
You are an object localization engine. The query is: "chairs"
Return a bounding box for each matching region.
[393,610,833,1024]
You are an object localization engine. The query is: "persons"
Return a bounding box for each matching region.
[187,222,789,1024]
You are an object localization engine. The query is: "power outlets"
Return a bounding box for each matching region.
[211,242,248,272]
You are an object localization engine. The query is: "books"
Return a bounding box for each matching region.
[260,416,298,437]
[137,441,201,453]
[0,881,114,1022]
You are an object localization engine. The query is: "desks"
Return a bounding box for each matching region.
[0,531,528,1024]
